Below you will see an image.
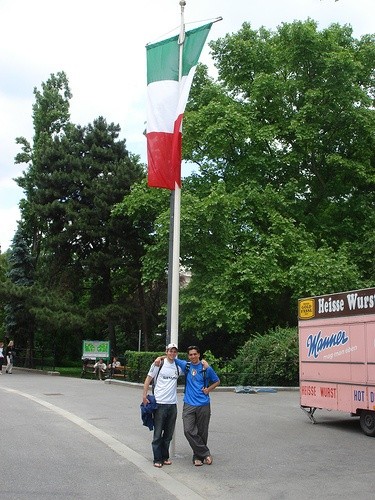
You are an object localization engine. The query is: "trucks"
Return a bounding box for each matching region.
[297,288,375,438]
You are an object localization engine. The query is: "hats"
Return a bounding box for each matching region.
[167,343,178,351]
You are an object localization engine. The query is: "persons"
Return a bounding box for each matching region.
[108,355,122,374]
[155,345,220,466]
[94,359,107,381]
[5,337,16,374]
[0,340,5,375]
[143,342,209,468]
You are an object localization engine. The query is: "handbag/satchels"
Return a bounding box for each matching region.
[10,359,13,363]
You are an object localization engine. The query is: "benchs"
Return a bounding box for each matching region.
[80,359,132,380]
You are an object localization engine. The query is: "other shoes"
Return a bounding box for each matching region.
[101,368,105,372]
[0,370,2,375]
[9,372,12,374]
[100,379,102,381]
[105,375,109,378]
[93,371,96,373]
[5,370,8,373]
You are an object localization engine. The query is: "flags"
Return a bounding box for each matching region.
[145,23,212,189]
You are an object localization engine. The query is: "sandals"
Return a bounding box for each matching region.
[163,459,172,465]
[154,460,163,468]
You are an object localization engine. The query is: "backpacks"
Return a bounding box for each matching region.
[152,359,179,395]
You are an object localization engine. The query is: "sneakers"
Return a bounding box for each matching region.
[206,455,213,465]
[195,459,203,466]
